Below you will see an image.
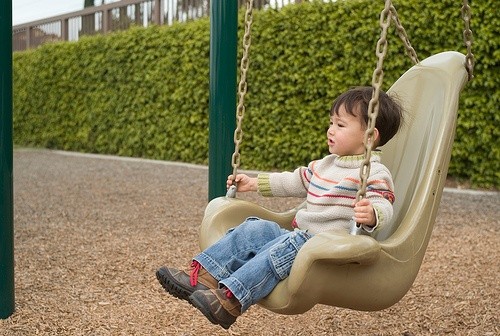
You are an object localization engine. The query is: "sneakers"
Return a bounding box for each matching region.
[156,261,219,307]
[189,289,241,330]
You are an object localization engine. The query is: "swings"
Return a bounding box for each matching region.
[198,0,477,316]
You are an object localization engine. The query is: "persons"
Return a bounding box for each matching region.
[156,87,403,331]
